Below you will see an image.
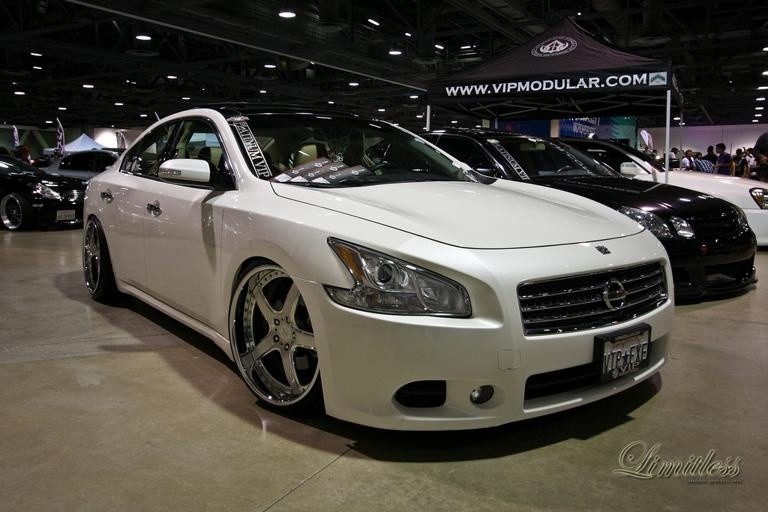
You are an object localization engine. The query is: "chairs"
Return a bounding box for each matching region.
[199,147,224,174]
[289,143,328,168]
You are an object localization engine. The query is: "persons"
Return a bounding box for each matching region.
[14,145,53,168]
[641,132,768,182]
